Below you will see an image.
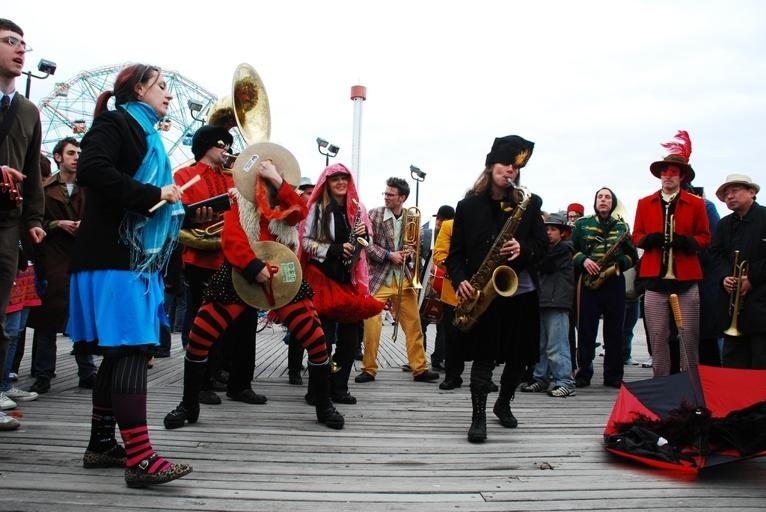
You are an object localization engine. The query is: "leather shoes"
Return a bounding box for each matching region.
[227,388,268,403]
[575,367,593,387]
[332,390,357,404]
[198,386,221,405]
[289,372,302,384]
[414,371,439,382]
[204,369,230,393]
[439,378,461,389]
[355,369,375,382]
[604,379,623,388]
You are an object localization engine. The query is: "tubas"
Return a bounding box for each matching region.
[178,62,271,251]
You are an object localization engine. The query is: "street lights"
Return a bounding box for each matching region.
[316,137,339,167]
[410,165,427,212]
[188,98,210,127]
[22,58,57,99]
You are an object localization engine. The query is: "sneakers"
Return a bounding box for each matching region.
[0,411,19,431]
[429,361,444,372]
[28,378,50,394]
[125,452,193,487]
[642,357,653,367]
[599,349,605,356]
[5,387,39,401]
[548,385,576,396]
[521,378,548,392]
[6,372,19,382]
[79,374,96,389]
[623,358,638,365]
[0,390,17,410]
[83,445,126,469]
[401,365,412,372]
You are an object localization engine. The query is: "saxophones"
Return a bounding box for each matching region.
[584,213,629,289]
[452,177,532,330]
[341,198,368,270]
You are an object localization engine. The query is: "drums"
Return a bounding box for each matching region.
[418,251,446,324]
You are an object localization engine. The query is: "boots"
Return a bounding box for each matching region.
[468,390,488,442]
[164,358,208,429]
[307,362,345,430]
[493,385,518,427]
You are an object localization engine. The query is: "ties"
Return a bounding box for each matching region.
[2,95,9,122]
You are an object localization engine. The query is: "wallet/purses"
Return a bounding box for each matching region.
[186,193,231,218]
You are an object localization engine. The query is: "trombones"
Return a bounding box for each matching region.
[392,206,423,343]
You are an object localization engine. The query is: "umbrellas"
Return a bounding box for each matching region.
[603,364,766,472]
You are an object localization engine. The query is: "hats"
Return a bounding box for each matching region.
[433,205,455,218]
[191,126,233,162]
[543,213,566,227]
[299,177,316,188]
[567,204,584,213]
[486,135,535,168]
[650,154,695,184]
[716,175,761,201]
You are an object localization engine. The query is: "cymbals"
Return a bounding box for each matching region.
[232,241,302,310]
[233,142,301,207]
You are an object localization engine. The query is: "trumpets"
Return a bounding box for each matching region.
[723,251,749,337]
[661,204,677,280]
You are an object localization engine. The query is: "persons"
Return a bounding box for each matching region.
[284,177,318,385]
[518,131,765,398]
[302,163,375,405]
[162,161,345,429]
[173,103,269,406]
[354,177,439,386]
[459,134,544,443]
[0,18,175,429]
[401,206,455,372]
[432,216,501,395]
[70,64,195,488]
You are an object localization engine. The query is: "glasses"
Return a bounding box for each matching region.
[0,36,32,53]
[382,192,400,197]
[568,215,579,220]
[214,140,231,150]
